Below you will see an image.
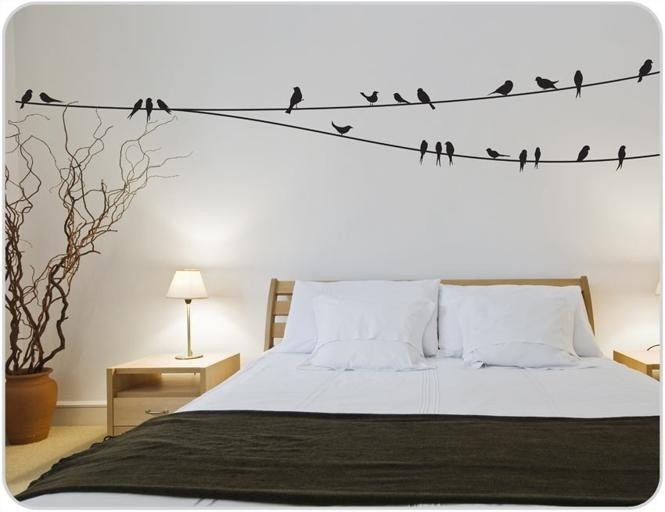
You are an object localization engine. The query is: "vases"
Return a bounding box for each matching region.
[8,367,62,449]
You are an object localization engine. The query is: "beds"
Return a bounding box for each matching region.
[16,273,657,506]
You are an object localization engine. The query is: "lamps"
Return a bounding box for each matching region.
[166,268,204,362]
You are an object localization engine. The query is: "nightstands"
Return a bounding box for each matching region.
[613,345,660,382]
[104,352,240,437]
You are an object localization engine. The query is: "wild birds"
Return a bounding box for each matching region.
[577,145,590,161]
[485,147,541,171]
[360,88,437,110]
[285,86,302,113]
[332,120,353,137]
[637,59,654,83]
[419,139,454,166]
[126,97,172,123]
[486,70,583,98]
[616,146,626,170]
[19,89,63,109]
